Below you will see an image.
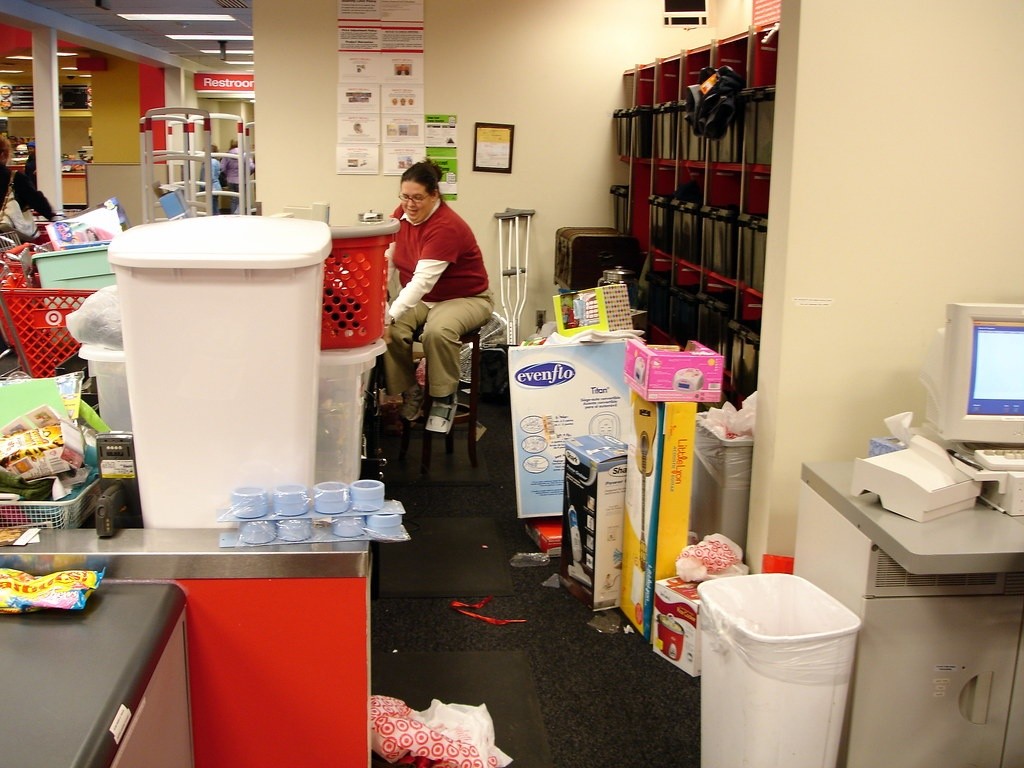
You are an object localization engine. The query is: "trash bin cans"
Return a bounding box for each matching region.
[697,573,861,768]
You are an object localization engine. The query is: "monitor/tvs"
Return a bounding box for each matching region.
[924,302,1024,454]
[282,202,330,226]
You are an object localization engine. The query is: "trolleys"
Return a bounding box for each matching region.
[0,211,99,430]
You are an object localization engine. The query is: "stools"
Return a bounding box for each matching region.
[398,317,480,469]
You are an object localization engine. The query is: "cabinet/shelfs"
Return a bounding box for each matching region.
[839,597,1024,768]
[139,106,256,223]
[630,19,781,409]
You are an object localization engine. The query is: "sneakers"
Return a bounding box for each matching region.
[400,384,425,422]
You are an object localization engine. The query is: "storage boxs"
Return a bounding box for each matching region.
[552,227,641,292]
[507,287,750,680]
[33,245,116,309]
[78,336,388,485]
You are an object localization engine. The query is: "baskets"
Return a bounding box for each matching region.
[0,477,100,530]
[2,223,100,377]
[320,218,402,351]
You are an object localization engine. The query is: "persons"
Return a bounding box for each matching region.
[24,142,38,188]
[384,161,496,435]
[397,64,409,75]
[220,138,255,215]
[0,135,13,210]
[355,124,363,134]
[200,145,222,215]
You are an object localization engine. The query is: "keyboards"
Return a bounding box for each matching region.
[973,449,1024,471]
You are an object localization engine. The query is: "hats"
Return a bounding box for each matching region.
[27,142,35,147]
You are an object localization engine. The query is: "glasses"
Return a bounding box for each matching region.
[398,191,433,203]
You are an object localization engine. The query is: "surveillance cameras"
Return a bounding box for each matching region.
[66,74,76,80]
